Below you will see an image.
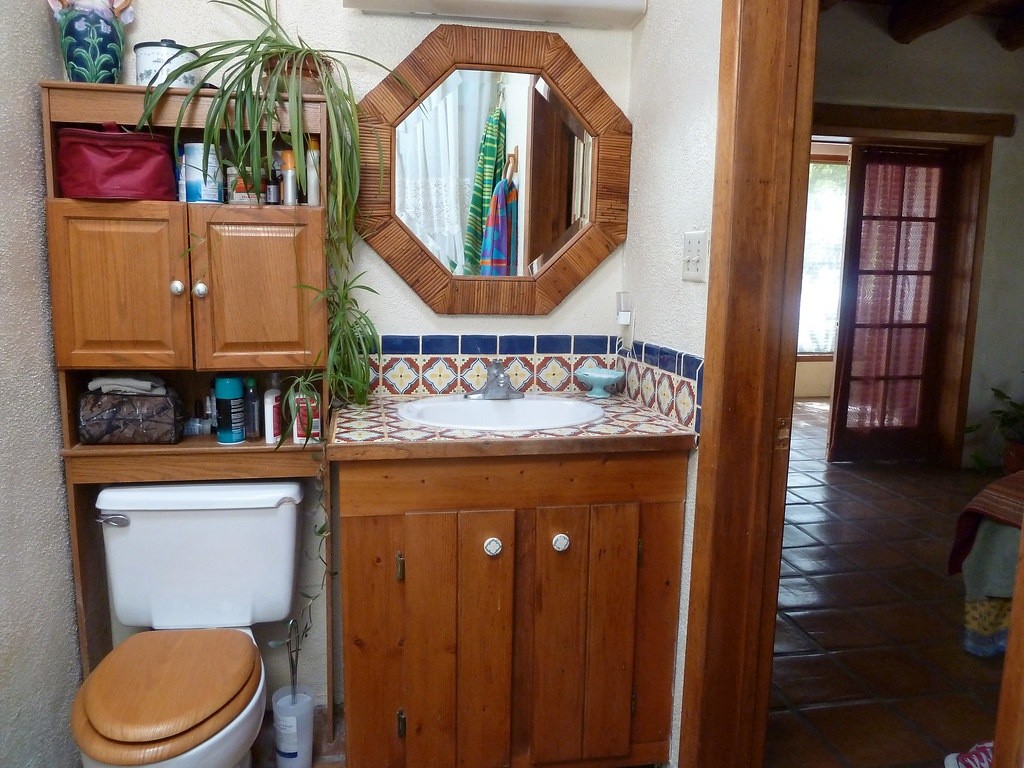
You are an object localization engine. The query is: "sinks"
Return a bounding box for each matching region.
[394,392,605,430]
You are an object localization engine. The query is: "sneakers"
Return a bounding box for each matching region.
[944,737,995,768]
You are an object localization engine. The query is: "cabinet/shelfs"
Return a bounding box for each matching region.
[37,79,330,484]
[341,450,687,768]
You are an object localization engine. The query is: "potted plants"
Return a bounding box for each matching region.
[959,387,1024,476]
[137,1,429,276]
[292,270,382,417]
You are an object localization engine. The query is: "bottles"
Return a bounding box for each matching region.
[206,377,246,445]
[244,378,261,442]
[183,417,211,436]
[176,136,321,207]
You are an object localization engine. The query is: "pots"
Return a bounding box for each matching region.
[133,39,202,90]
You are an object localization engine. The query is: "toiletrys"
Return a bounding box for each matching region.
[262,374,320,443]
[266,133,319,206]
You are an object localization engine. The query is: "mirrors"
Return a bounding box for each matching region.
[352,24,632,315]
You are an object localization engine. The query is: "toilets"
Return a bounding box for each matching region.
[70,481,304,768]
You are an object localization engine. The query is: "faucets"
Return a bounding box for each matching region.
[464,358,525,399]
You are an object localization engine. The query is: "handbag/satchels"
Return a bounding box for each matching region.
[56,118,177,201]
[77,376,185,445]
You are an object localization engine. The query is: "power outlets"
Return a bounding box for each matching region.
[681,230,704,283]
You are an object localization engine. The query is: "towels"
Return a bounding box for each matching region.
[461,107,519,275]
